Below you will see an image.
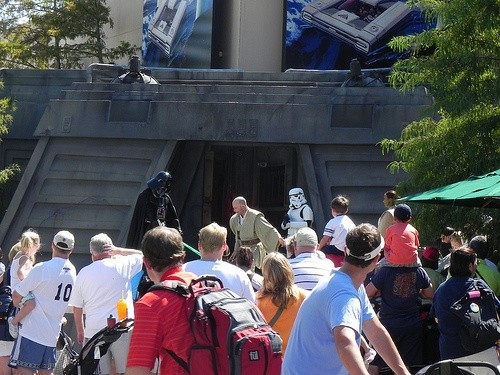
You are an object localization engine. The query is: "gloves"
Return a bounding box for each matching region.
[281,214,290,227]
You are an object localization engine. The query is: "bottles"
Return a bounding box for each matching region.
[107,315,115,334]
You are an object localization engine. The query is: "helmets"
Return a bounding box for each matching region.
[146,170,172,188]
[288,188,307,209]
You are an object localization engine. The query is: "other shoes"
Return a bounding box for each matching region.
[8,316,18,339]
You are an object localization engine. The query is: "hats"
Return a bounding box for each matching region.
[421,246,439,261]
[53,230,74,250]
[394,204,412,218]
[443,227,454,236]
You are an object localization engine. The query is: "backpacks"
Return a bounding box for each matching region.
[146,274,283,375]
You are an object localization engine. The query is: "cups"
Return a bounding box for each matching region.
[116,298,128,327]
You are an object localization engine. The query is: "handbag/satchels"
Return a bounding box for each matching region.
[447,286,500,353]
[53,342,71,375]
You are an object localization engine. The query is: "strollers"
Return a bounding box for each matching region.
[52,314,134,375]
[414,359,500,375]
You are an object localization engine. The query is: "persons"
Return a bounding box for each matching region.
[183,221,256,309]
[73,232,144,375]
[281,187,313,237]
[281,222,412,375]
[256,251,311,365]
[137,171,183,299]
[124,226,214,375]
[317,188,500,375]
[0,228,40,339]
[16,230,76,375]
[227,196,285,293]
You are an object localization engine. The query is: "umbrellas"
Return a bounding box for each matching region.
[393,169,500,200]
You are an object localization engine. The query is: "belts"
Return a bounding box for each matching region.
[241,239,260,245]
